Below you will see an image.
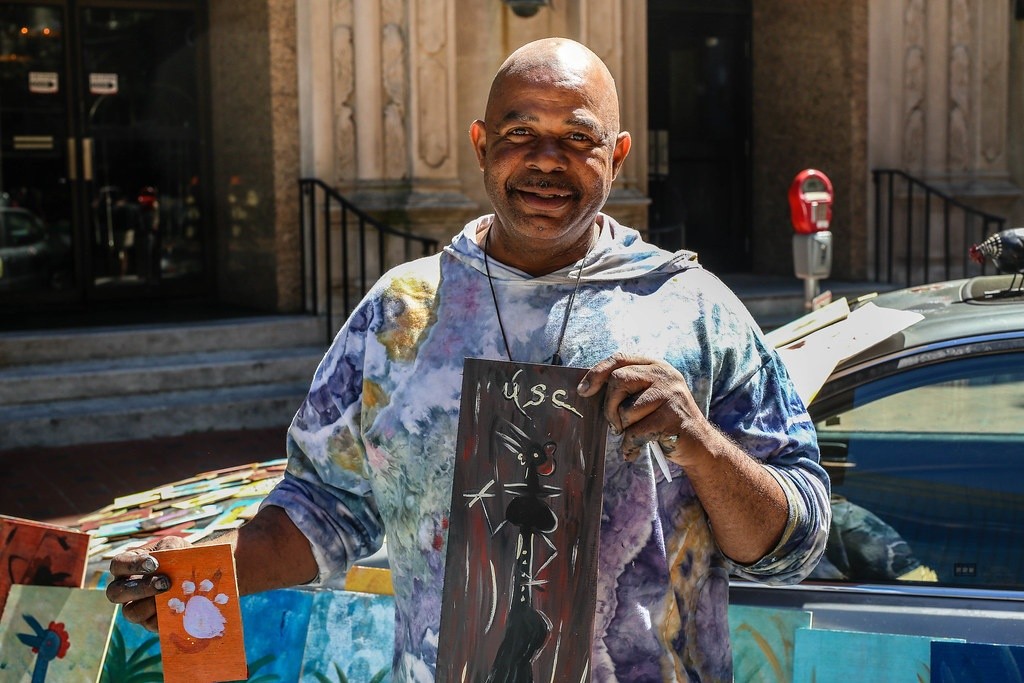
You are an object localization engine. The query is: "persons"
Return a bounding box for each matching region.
[104,34,833,683]
[801,441,932,583]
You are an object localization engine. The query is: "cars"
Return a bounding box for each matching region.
[728,227,1024,648]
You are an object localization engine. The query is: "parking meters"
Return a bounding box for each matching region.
[789,168,833,312]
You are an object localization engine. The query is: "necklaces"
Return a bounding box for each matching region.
[484,223,589,363]
